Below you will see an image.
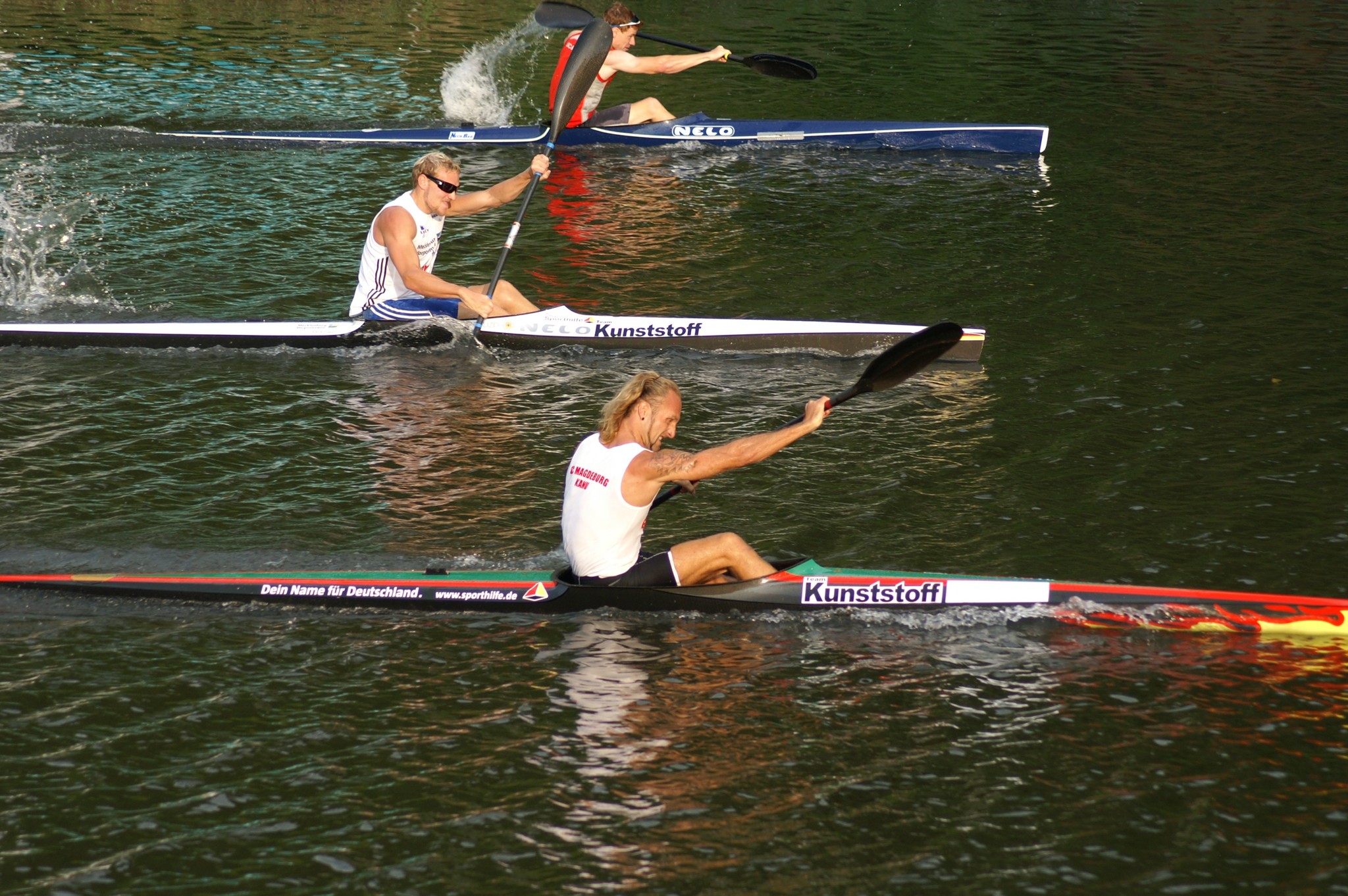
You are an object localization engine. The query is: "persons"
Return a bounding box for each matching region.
[549,2,731,130]
[563,371,832,587]
[348,152,550,322]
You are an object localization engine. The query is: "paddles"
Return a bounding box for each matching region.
[650,321,964,507]
[470,19,614,338]
[535,1,817,81]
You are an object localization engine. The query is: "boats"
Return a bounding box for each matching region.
[153,115,1051,158]
[0,554,1348,641]
[0,306,987,365]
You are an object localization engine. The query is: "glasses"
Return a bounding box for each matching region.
[608,14,640,26]
[425,171,458,195]
